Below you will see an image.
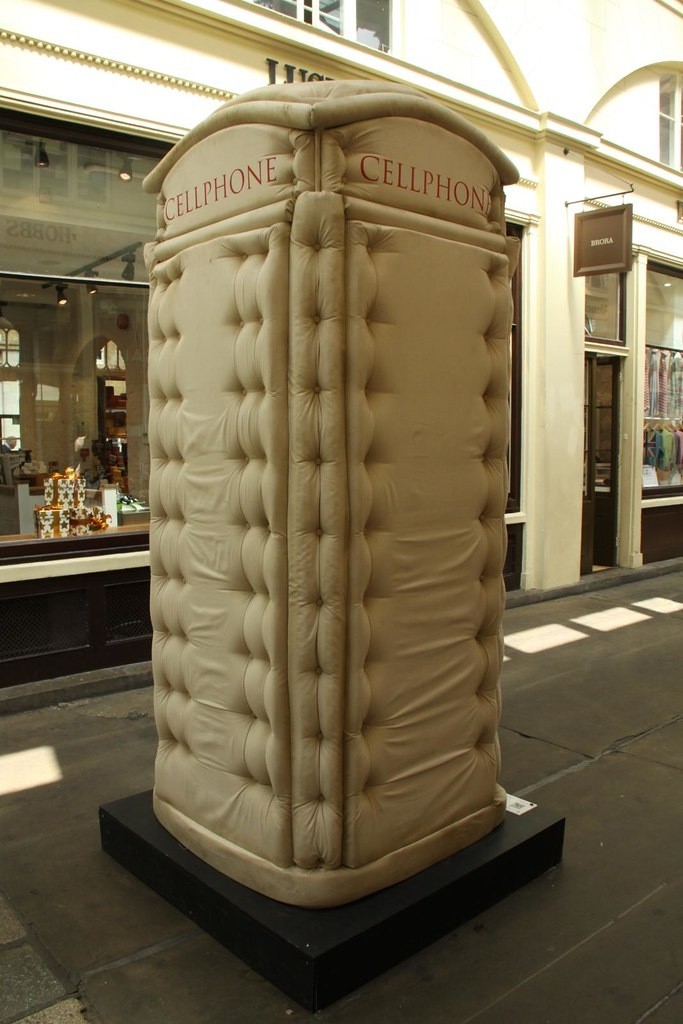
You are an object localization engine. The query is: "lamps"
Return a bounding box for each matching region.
[85,268,99,294]
[118,152,133,183]
[55,282,68,304]
[121,250,136,281]
[35,141,50,168]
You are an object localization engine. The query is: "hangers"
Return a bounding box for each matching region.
[644,422,683,431]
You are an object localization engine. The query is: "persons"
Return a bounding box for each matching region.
[75,436,100,476]
[1,436,17,453]
